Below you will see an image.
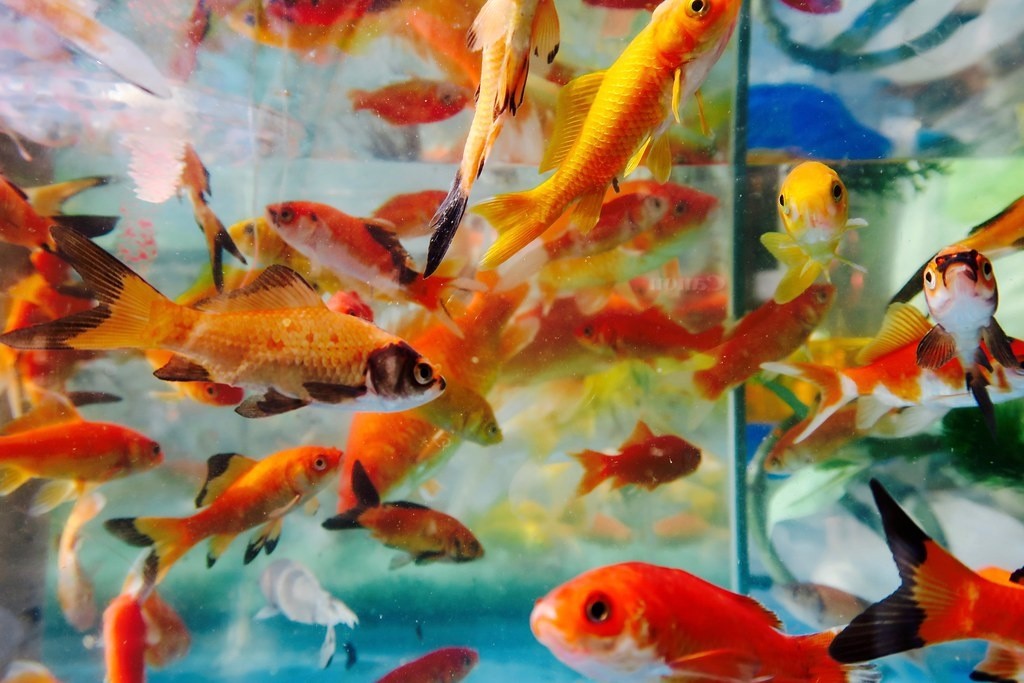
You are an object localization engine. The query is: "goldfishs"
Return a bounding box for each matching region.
[1,1,1024,683]
